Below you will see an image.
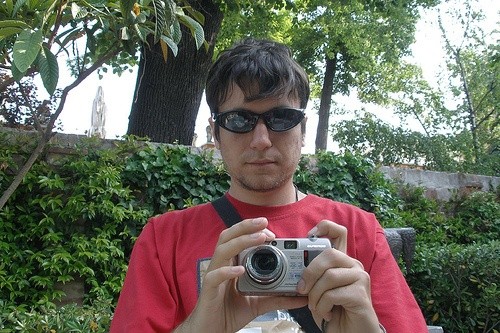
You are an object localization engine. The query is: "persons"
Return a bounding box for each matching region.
[109,39,429,333]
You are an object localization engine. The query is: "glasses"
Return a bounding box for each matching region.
[212,109,304,133]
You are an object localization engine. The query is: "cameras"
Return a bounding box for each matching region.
[236,237,332,296]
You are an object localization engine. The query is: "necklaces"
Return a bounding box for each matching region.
[293,183,299,204]
